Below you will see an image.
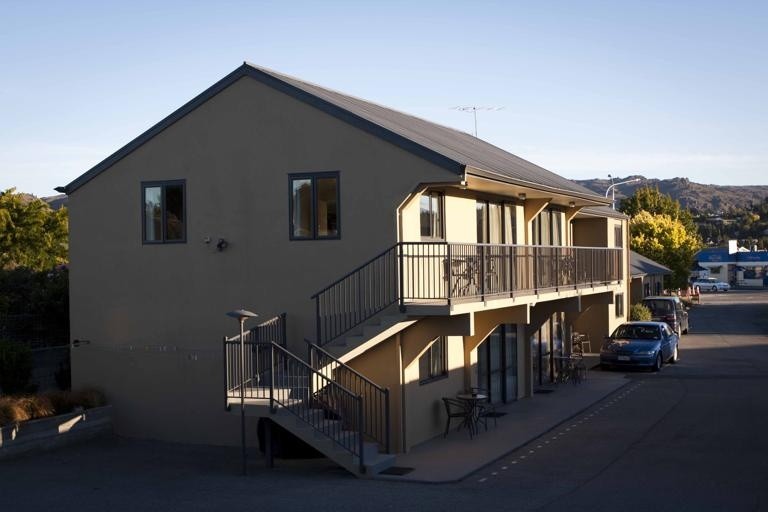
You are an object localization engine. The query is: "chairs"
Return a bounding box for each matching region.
[442,387,496,440]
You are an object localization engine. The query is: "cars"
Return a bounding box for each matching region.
[600,321,680,372]
[693,278,731,292]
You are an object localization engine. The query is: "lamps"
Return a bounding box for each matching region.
[519,193,526,200]
[569,202,575,208]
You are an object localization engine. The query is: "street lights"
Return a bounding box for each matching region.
[604,174,640,209]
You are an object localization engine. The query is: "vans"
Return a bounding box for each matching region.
[643,295,688,338]
